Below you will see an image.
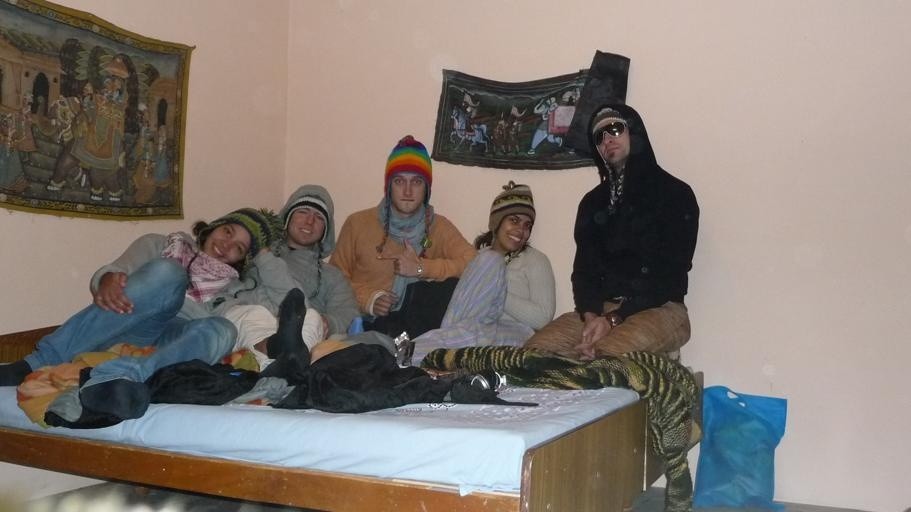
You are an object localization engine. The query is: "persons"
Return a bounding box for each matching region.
[239,182,357,387]
[521,102,700,380]
[395,178,559,376]
[325,133,475,357]
[527,96,567,155]
[0,204,288,420]
[505,105,521,154]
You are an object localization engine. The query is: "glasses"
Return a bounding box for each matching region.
[593,121,626,146]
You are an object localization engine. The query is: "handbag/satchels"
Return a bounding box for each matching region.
[692,385,787,510]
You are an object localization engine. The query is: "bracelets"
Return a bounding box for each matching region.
[604,310,620,329]
[415,256,423,279]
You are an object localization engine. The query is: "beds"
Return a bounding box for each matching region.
[2,325,707,510]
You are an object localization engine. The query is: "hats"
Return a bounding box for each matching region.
[282,196,329,243]
[592,108,624,134]
[384,135,432,201]
[489,180,536,234]
[211,209,282,259]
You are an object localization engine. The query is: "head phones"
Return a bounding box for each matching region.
[469,368,502,392]
